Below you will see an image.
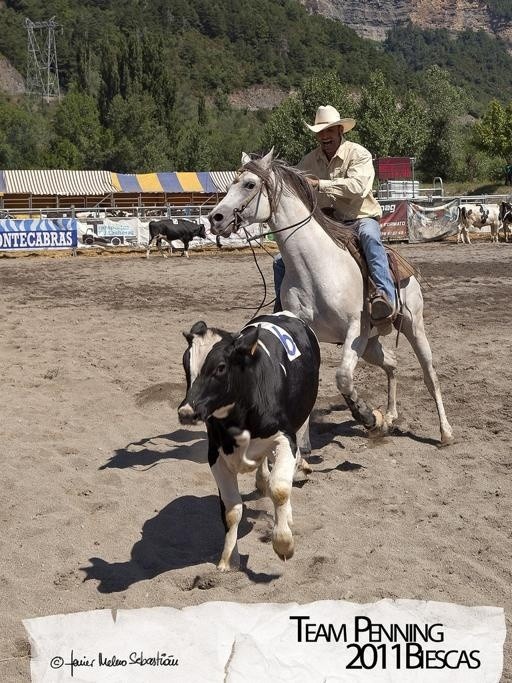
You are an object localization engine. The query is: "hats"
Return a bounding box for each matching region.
[302,103,357,137]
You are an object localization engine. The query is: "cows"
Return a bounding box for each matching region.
[176,309,322,573]
[145,217,206,260]
[452,201,512,244]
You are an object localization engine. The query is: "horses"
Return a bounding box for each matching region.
[207,144,456,445]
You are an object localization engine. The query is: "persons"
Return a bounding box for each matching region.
[272,104,398,339]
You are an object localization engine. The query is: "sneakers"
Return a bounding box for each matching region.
[369,298,395,337]
